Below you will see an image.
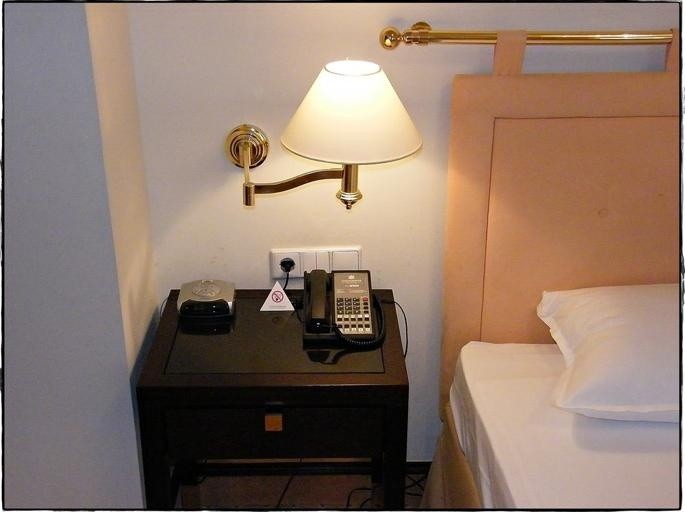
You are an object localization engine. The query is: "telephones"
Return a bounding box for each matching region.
[302,267,387,350]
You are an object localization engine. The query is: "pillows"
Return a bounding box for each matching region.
[535,280,681,424]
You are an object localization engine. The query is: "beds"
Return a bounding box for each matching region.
[378,16,684,510]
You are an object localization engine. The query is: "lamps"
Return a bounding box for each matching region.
[224,59,424,212]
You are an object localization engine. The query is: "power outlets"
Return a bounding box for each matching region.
[269,248,304,279]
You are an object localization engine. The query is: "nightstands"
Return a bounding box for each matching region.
[131,286,411,510]
